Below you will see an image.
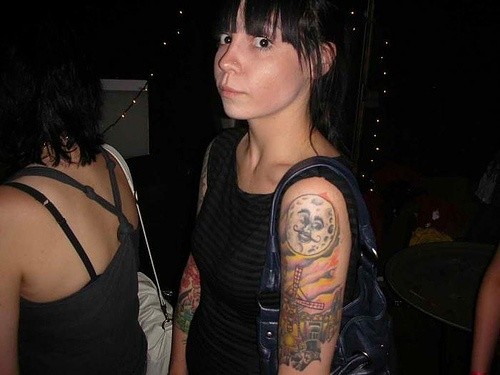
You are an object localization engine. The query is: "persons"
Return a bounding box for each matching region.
[0,37,147,375]
[315,73,498,374]
[167,2,367,374]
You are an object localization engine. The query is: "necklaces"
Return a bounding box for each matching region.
[41,146,77,161]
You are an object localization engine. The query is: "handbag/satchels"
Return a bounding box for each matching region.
[134,270,173,374]
[256,157,390,375]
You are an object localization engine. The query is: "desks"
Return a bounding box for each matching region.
[385,243,500,375]
